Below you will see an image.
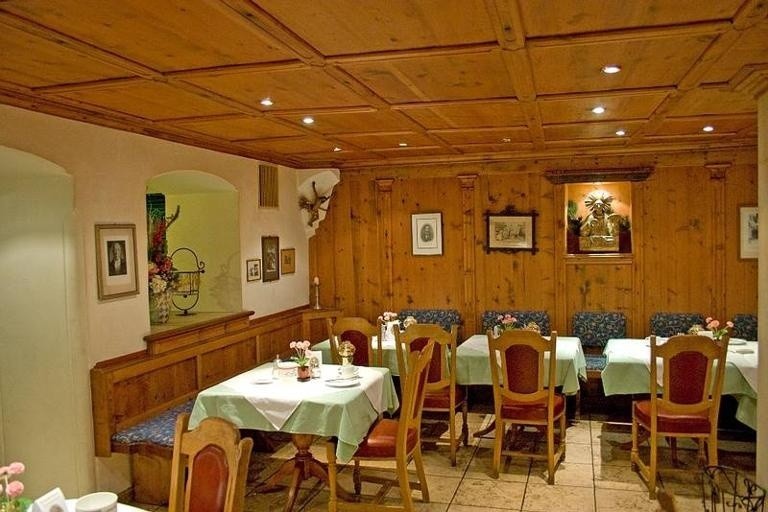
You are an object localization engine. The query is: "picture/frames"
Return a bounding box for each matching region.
[246,259,260,282]
[261,235,279,282]
[280,247,295,275]
[409,211,442,256]
[483,204,540,256]
[736,203,757,262]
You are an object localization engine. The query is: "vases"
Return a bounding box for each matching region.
[94,222,139,301]
[298,365,310,381]
[148,291,172,324]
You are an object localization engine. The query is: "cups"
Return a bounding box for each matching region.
[74,490,118,511]
[494,325,501,337]
[645,336,659,345]
[341,365,355,378]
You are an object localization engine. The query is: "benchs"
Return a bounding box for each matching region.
[112,400,198,506]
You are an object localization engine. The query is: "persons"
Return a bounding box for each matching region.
[581,198,615,236]
[109,241,126,275]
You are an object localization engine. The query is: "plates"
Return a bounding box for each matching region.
[727,337,754,354]
[324,376,360,387]
[256,362,296,383]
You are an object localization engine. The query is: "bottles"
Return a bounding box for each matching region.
[271,353,282,379]
[381,320,387,342]
[310,356,320,379]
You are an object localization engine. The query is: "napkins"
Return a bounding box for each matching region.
[326,366,384,414]
[231,383,308,430]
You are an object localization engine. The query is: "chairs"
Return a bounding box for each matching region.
[701,466,766,512]
[352,338,435,512]
[730,313,758,341]
[572,311,626,423]
[168,413,253,512]
[631,334,729,503]
[650,313,704,338]
[397,310,462,346]
[326,317,383,367]
[393,324,469,466]
[487,330,566,485]
[481,311,550,335]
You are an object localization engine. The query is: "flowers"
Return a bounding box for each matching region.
[145,202,184,293]
[378,312,397,322]
[498,315,517,329]
[1,462,29,512]
[705,317,734,340]
[290,340,310,366]
